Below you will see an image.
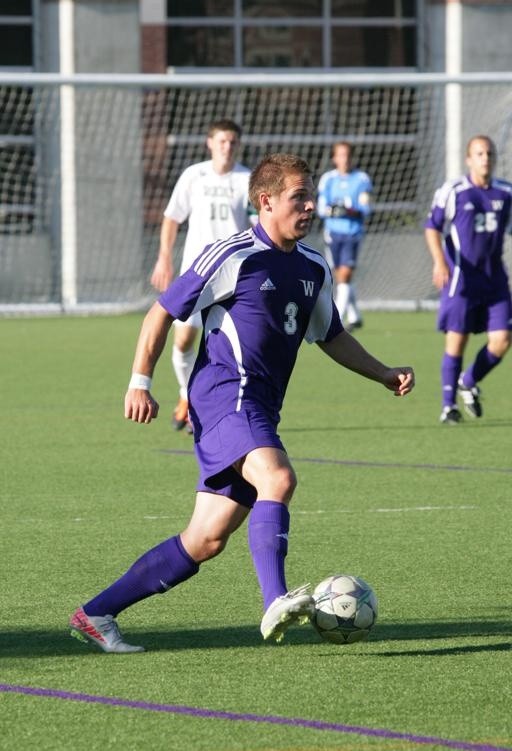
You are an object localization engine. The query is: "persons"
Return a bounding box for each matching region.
[316,141,374,333]
[425,135,512,425]
[67,155,415,653]
[150,121,259,435]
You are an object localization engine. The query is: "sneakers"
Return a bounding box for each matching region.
[456,371,483,420]
[345,320,362,335]
[68,604,148,655]
[438,404,462,425]
[259,581,317,641]
[169,395,190,432]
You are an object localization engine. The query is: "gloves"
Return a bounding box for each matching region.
[325,203,346,218]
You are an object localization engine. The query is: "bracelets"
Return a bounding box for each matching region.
[129,372,152,391]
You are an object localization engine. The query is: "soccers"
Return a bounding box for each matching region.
[309,575,378,643]
